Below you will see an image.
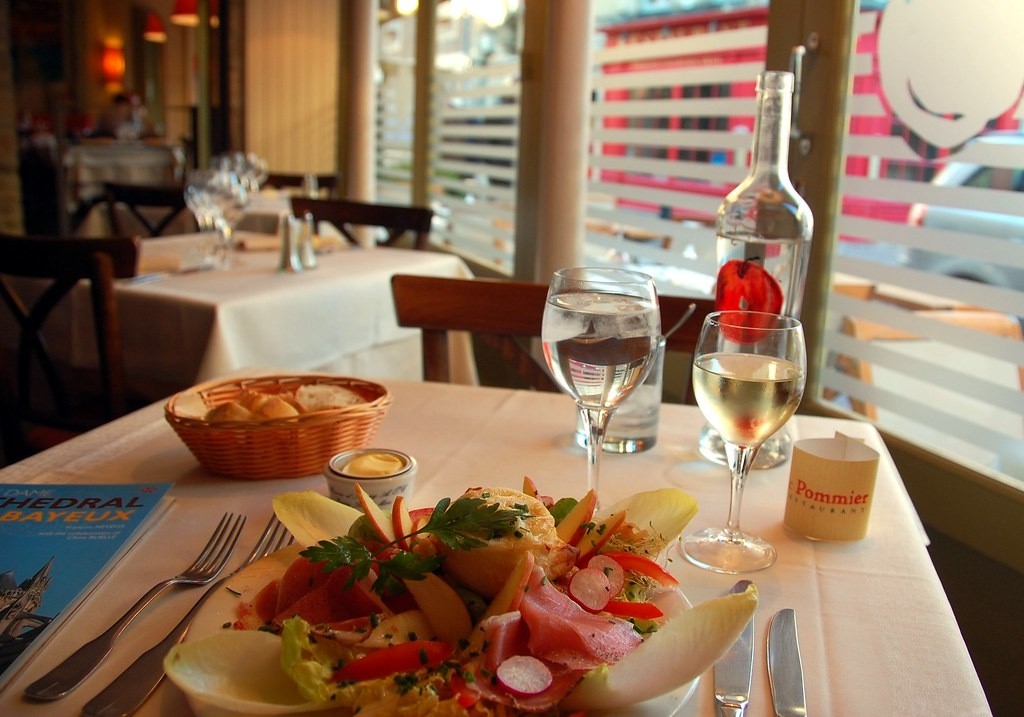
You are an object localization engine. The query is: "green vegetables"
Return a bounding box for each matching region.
[159,488,760,717]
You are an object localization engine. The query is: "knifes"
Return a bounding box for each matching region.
[765,608,808,717]
[713,579,755,717]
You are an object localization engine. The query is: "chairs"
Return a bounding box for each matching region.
[0,225,144,461]
[67,176,197,246]
[380,270,836,403]
[286,192,438,248]
[247,166,344,236]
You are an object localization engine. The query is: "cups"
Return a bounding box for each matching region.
[574,333,667,455]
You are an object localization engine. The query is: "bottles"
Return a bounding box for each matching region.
[300,211,316,268]
[278,215,303,275]
[697,69,816,474]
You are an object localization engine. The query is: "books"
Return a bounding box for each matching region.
[0,482,179,700]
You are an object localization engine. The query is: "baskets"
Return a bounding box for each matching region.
[163,374,393,481]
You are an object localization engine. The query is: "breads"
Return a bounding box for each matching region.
[428,488,580,598]
[204,383,368,421]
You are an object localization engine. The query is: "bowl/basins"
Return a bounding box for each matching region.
[323,448,419,515]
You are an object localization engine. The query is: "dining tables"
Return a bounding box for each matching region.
[0,374,991,717]
[0,227,477,397]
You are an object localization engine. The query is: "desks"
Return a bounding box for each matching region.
[63,146,174,227]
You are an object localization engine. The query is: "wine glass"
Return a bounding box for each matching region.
[540,267,662,526]
[183,150,268,266]
[679,310,808,575]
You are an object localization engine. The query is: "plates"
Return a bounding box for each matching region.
[187,544,701,716]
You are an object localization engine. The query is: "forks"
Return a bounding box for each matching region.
[81,511,295,717]
[24,511,247,704]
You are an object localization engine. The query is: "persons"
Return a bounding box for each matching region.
[111,94,167,147]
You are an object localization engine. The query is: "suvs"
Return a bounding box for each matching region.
[899,133,1024,294]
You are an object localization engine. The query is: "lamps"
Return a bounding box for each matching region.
[169,0,219,28]
[142,12,167,42]
